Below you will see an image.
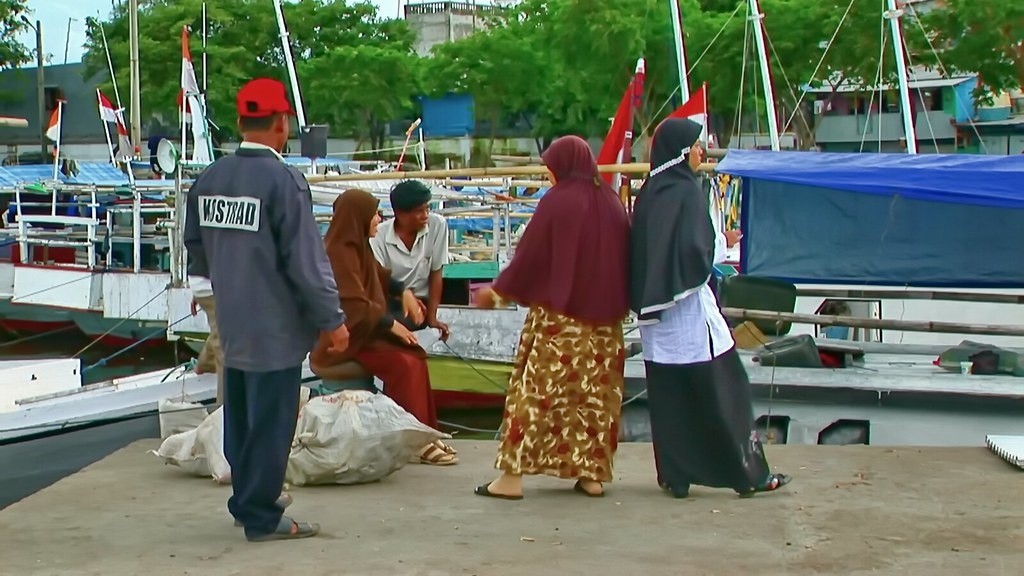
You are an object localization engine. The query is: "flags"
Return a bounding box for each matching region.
[178,27,199,134]
[97,93,135,157]
[44,104,61,143]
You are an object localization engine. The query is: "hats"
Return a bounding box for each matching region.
[237,79,297,118]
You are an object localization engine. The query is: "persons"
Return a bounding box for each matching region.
[309,180,459,466]
[629,117,792,502]
[473,136,629,501]
[183,78,349,542]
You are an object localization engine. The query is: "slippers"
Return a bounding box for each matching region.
[234,494,292,526]
[575,479,605,497]
[246,516,321,541]
[474,481,523,500]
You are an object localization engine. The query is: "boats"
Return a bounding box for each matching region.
[0,0,1024,450]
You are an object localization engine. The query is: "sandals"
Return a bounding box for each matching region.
[740,474,793,498]
[660,481,689,497]
[410,443,459,464]
[435,439,458,454]
